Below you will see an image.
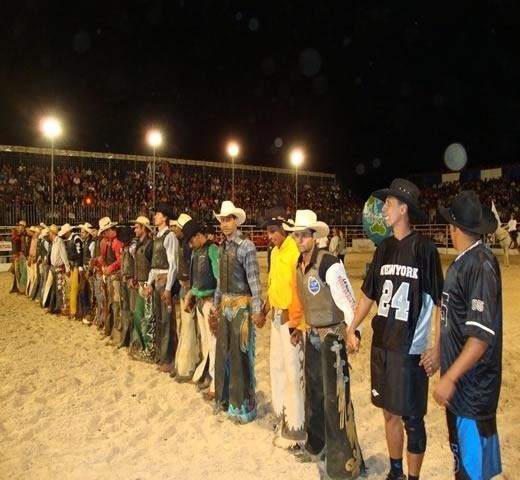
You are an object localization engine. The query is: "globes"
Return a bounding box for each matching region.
[362,188,395,248]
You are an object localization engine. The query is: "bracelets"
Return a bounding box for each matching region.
[259,311,268,319]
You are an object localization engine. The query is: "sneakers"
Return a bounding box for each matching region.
[385,472,406,480]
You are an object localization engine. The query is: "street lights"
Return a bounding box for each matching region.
[38,114,66,224]
[144,125,169,226]
[222,139,242,231]
[286,142,307,232]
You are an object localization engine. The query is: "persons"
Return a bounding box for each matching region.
[280,207,368,480]
[344,175,445,480]
[416,187,504,480]
[252,204,310,452]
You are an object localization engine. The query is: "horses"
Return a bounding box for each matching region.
[495,227,520,264]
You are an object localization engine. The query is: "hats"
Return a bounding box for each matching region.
[212,201,246,227]
[373,178,427,221]
[181,221,204,244]
[89,225,99,230]
[255,209,294,229]
[436,189,500,236]
[11,220,57,238]
[97,216,118,236]
[58,223,76,237]
[149,203,172,218]
[77,221,93,235]
[176,213,192,229]
[116,226,137,243]
[283,210,330,240]
[129,215,152,233]
